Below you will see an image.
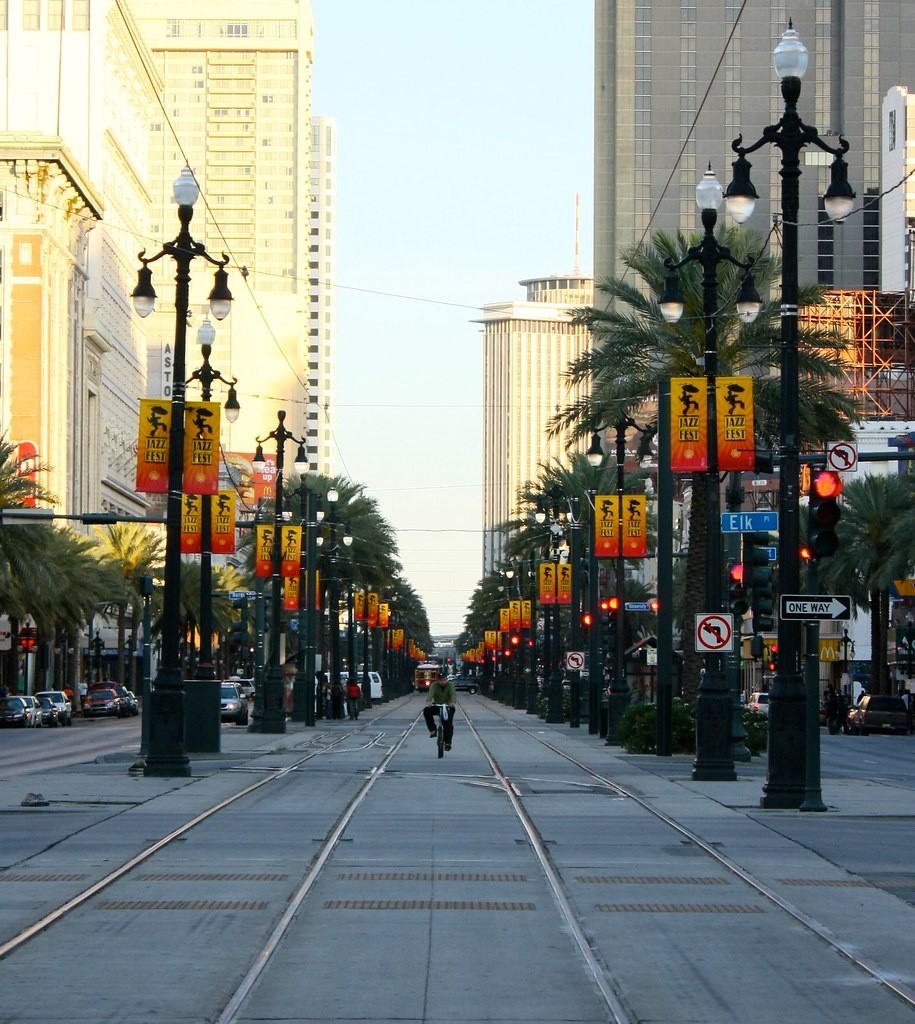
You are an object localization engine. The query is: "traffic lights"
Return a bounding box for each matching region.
[741,534,771,590]
[807,470,841,559]
[607,599,618,635]
[749,636,765,658]
[728,562,750,617]
[751,565,777,634]
[769,643,779,672]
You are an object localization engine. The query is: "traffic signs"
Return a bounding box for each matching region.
[778,593,852,621]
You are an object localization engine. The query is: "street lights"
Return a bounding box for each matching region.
[252,408,312,733]
[716,16,859,808]
[313,485,355,717]
[836,621,856,711]
[185,307,244,682]
[897,612,915,694]
[128,155,240,775]
[489,545,555,721]
[16,617,38,696]
[534,483,572,724]
[584,411,659,747]
[87,624,107,681]
[52,628,75,691]
[653,158,768,782]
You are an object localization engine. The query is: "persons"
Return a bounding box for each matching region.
[81,680,87,698]
[423,673,456,751]
[321,676,361,719]
[50,684,73,699]
[823,687,845,734]
[857,690,870,703]
[0,684,11,698]
[902,689,914,710]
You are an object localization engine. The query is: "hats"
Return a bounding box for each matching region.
[438,674,447,678]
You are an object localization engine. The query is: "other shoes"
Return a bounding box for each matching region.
[430,729,437,738]
[349,716,359,721]
[445,742,451,752]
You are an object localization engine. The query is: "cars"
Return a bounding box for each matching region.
[314,670,382,715]
[446,672,480,695]
[219,677,256,726]
[0,680,140,728]
[740,692,769,716]
[846,693,909,735]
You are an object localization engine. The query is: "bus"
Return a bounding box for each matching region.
[412,661,441,693]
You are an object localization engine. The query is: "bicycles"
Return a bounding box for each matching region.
[426,701,454,759]
